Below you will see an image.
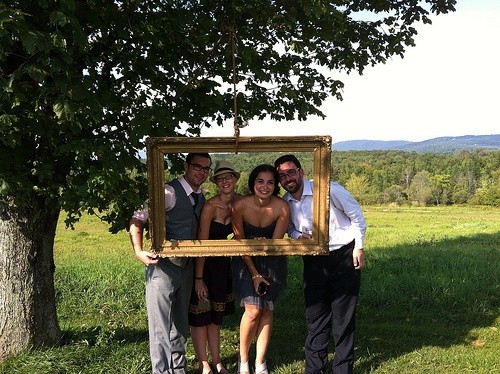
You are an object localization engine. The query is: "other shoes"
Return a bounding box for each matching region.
[237,354,250,374]
[254,359,269,374]
[198,366,213,374]
[211,362,229,374]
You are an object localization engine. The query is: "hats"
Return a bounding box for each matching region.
[209,161,240,183]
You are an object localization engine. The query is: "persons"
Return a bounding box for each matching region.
[129,152,213,374]
[189,161,244,374]
[231,164,290,374]
[275,154,366,374]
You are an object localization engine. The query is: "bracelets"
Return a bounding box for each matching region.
[252,275,262,280]
[196,278,203,279]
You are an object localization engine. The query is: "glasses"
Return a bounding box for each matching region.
[279,167,299,180]
[188,162,213,174]
[214,174,235,182]
